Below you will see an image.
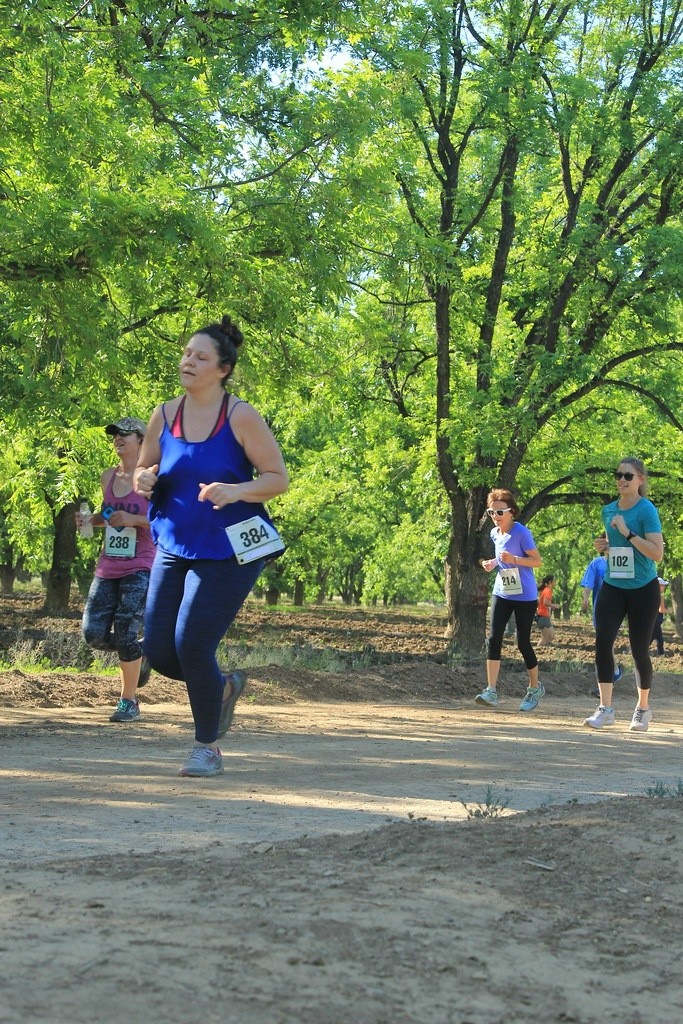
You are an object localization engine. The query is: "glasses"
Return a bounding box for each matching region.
[486,508,511,517]
[111,430,139,439]
[613,471,642,481]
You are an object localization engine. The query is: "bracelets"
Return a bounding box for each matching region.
[514,556,517,563]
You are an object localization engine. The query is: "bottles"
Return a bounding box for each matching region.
[79,498,94,539]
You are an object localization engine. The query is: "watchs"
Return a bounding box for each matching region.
[626,530,636,540]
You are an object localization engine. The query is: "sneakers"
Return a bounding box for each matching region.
[137,636,151,688]
[218,670,245,738]
[109,699,142,721]
[584,705,615,728]
[475,687,498,707]
[179,743,224,777]
[521,681,545,711]
[630,704,653,730]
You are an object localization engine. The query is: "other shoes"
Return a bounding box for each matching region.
[597,665,622,696]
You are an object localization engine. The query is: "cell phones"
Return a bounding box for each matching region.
[101,506,125,532]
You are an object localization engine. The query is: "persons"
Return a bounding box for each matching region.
[649,577,669,657]
[536,575,559,646]
[583,457,663,732]
[474,489,545,711]
[580,533,622,698]
[133,315,288,777]
[74,417,152,722]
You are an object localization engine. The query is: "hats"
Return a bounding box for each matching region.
[105,417,146,439]
